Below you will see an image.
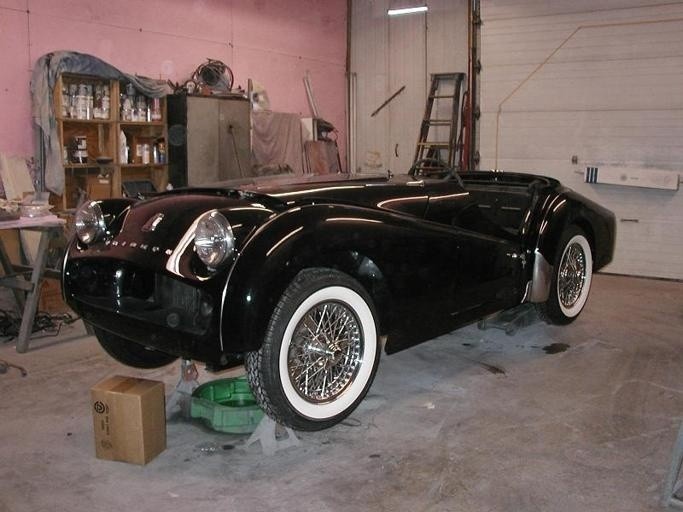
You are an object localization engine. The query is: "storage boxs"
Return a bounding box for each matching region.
[37,276,63,314]
[90,375,168,466]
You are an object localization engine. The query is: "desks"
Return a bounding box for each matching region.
[0,214,65,353]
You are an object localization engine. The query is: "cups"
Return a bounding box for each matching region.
[79,82,92,95]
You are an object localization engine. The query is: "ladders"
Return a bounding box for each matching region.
[412,72,464,174]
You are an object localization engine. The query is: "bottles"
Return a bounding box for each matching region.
[141,137,166,164]
[175,352,200,419]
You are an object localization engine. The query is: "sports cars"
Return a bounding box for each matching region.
[59,156,616,432]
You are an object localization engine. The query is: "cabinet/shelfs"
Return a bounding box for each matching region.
[38,70,168,212]
[167,96,251,187]
